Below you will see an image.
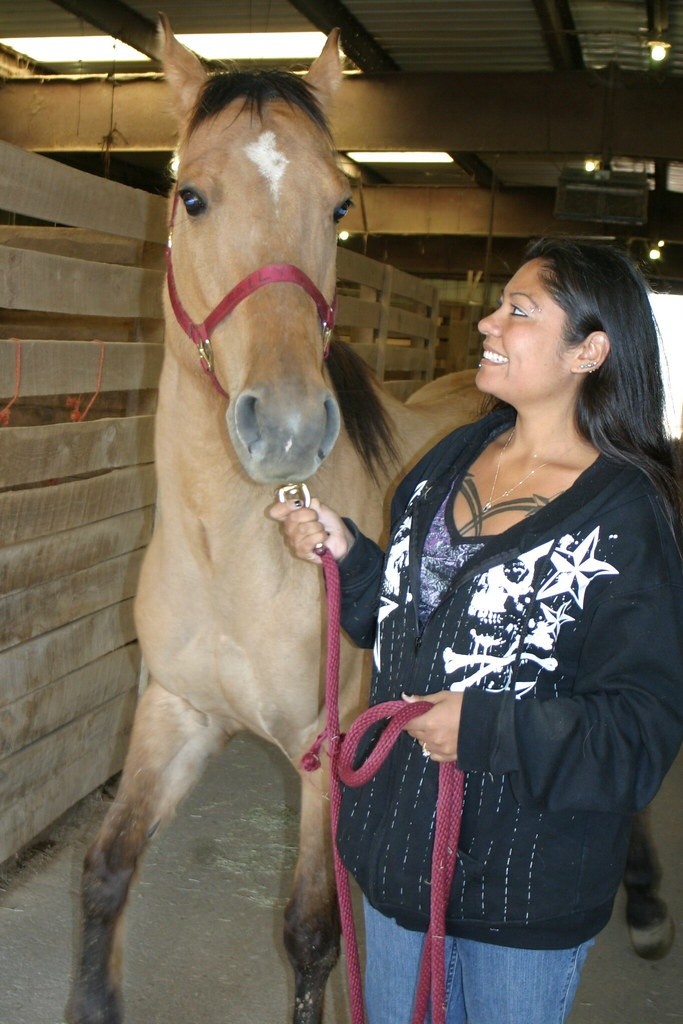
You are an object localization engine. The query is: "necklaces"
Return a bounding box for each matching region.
[482,428,578,514]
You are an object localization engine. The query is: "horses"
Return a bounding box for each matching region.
[62,11,673,1024]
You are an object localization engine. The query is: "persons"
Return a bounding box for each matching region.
[268,237,683,1023]
